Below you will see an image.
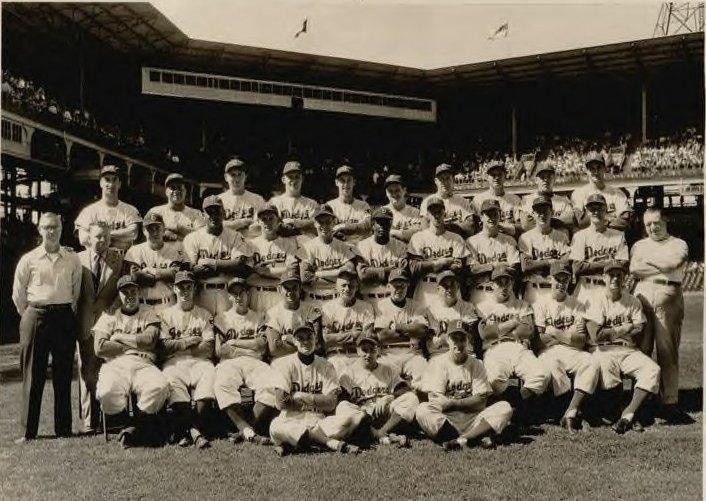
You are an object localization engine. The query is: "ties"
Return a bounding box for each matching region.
[93,257,101,293]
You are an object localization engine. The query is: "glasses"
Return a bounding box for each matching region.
[41,225,60,230]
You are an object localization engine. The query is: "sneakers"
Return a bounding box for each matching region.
[479,436,492,448]
[560,417,577,435]
[195,435,209,451]
[227,433,240,444]
[633,421,642,433]
[250,434,270,446]
[397,434,408,449]
[611,418,629,435]
[336,442,347,453]
[178,437,192,447]
[442,439,461,451]
[273,445,287,458]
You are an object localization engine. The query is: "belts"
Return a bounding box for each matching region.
[421,275,439,284]
[383,342,415,351]
[638,278,680,287]
[308,292,339,300]
[359,395,384,405]
[303,407,321,413]
[32,302,73,312]
[250,285,278,292]
[582,277,606,286]
[139,293,177,306]
[327,346,357,359]
[356,290,392,300]
[522,281,554,290]
[125,350,155,363]
[471,282,494,293]
[487,337,526,349]
[594,339,630,348]
[198,280,228,292]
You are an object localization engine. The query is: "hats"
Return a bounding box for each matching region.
[434,163,453,177]
[202,195,223,209]
[292,320,316,337]
[224,276,249,292]
[445,320,470,339]
[283,161,303,176]
[491,264,515,282]
[602,260,623,272]
[480,199,501,215]
[531,196,551,209]
[585,194,607,208]
[255,203,279,217]
[164,173,185,188]
[98,164,121,179]
[312,204,335,219]
[171,270,196,286]
[337,265,359,279]
[425,196,445,210]
[383,174,406,189]
[372,208,395,222]
[386,268,408,282]
[584,152,604,165]
[336,165,354,179]
[224,159,247,174]
[114,274,140,288]
[433,269,459,286]
[487,161,506,173]
[141,212,163,226]
[549,261,574,278]
[277,268,301,286]
[535,163,555,177]
[355,332,380,348]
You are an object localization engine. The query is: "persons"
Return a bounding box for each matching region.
[427,270,478,361]
[321,271,375,358]
[588,130,629,176]
[143,173,203,242]
[406,196,471,308]
[477,263,550,408]
[374,268,429,382]
[12,212,83,443]
[571,151,631,230]
[159,270,215,449]
[217,159,264,221]
[533,261,599,436]
[354,208,408,302]
[628,128,706,181]
[183,195,253,316]
[528,130,588,182]
[327,166,372,249]
[76,221,124,434]
[520,164,574,241]
[376,175,423,242]
[90,274,168,447]
[628,205,689,423]
[464,199,520,320]
[340,336,419,447]
[265,273,322,356]
[245,202,299,316]
[583,260,661,435]
[518,195,570,310]
[419,163,474,238]
[2,80,95,129]
[569,194,629,306]
[75,166,142,253]
[456,130,527,182]
[268,162,319,249]
[213,277,275,444]
[416,319,514,453]
[125,213,191,307]
[473,162,521,237]
[269,325,360,457]
[300,204,356,305]
